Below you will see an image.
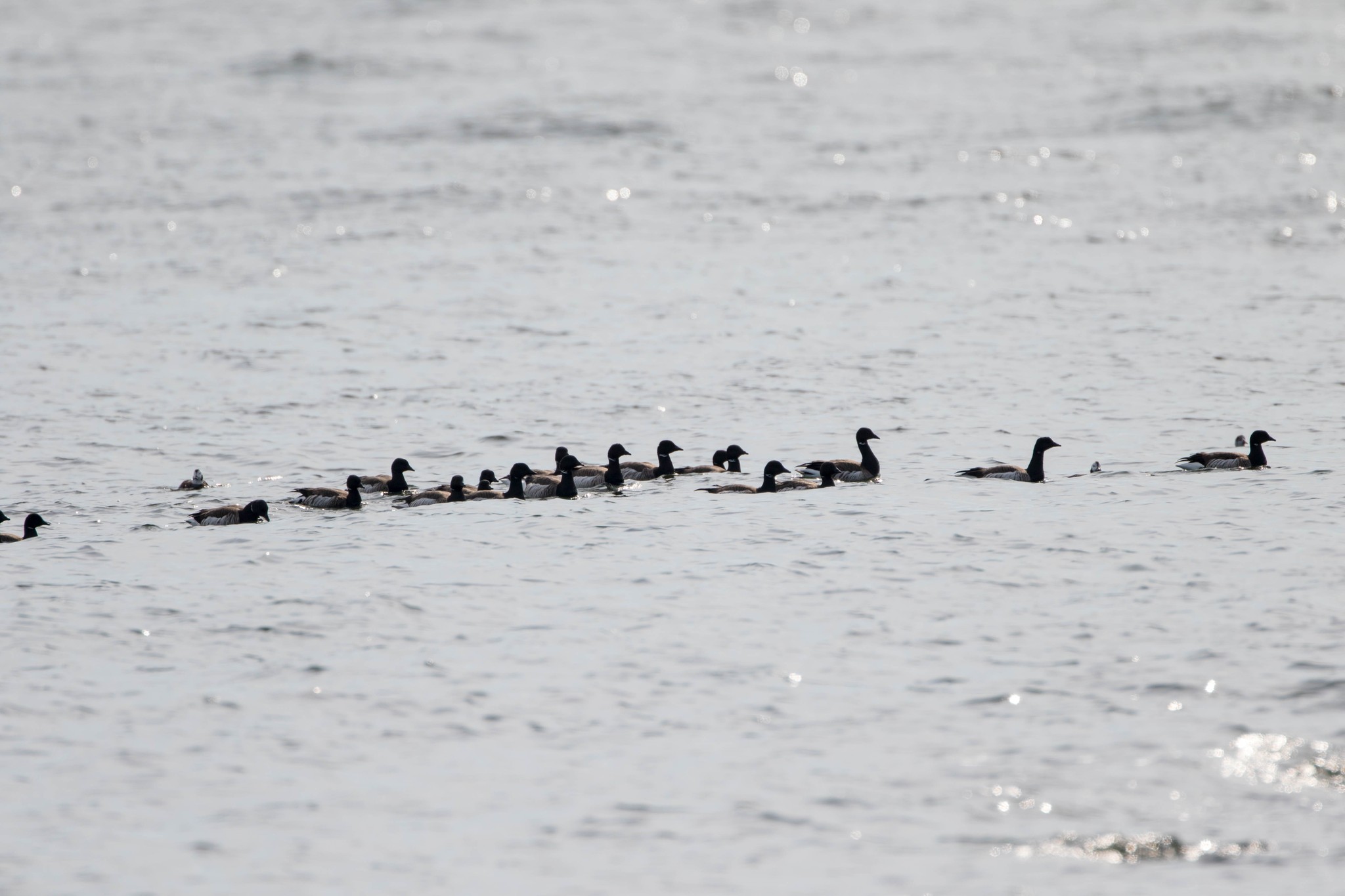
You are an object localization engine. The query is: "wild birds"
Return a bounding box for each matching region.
[171,427,880,527]
[954,436,1061,482]
[0,510,50,543]
[1176,430,1276,471]
[1063,461,1102,478]
